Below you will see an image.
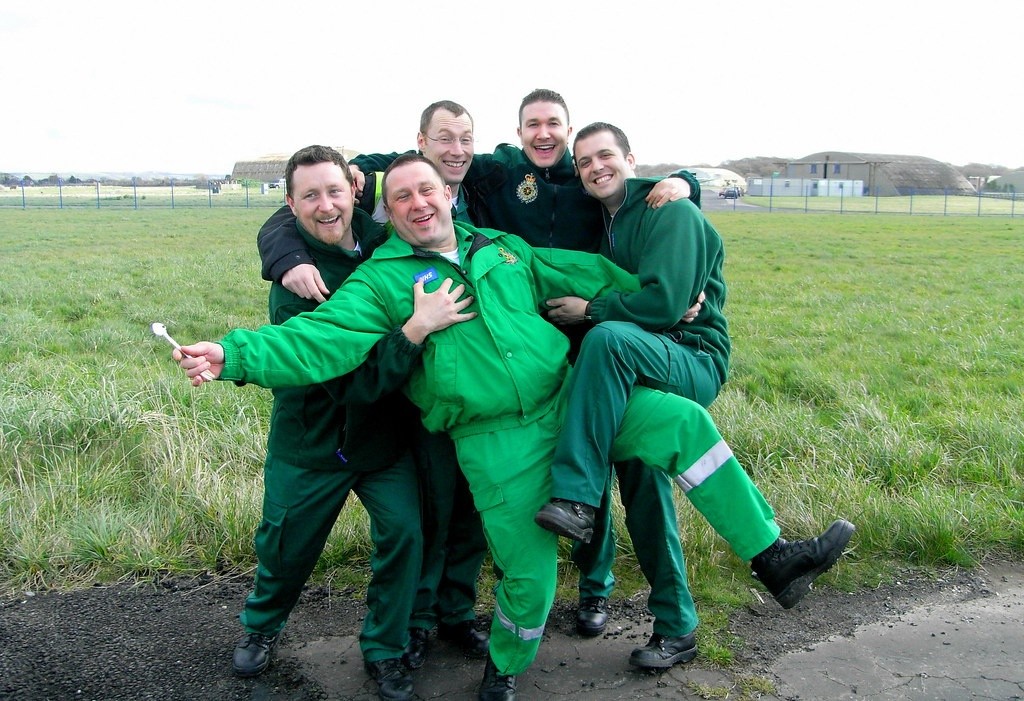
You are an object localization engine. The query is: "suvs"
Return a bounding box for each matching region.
[269,178,286,189]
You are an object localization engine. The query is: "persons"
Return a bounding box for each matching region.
[173,89,855,701]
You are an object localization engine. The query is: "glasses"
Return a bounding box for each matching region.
[425,133,474,147]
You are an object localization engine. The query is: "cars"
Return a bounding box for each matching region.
[719,186,744,199]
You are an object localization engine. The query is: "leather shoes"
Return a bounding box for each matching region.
[365,651,416,701]
[751,520,855,609]
[401,628,428,668]
[535,498,595,545]
[233,630,282,675]
[630,634,697,667]
[576,594,606,632]
[437,621,491,656]
[478,653,517,701]
